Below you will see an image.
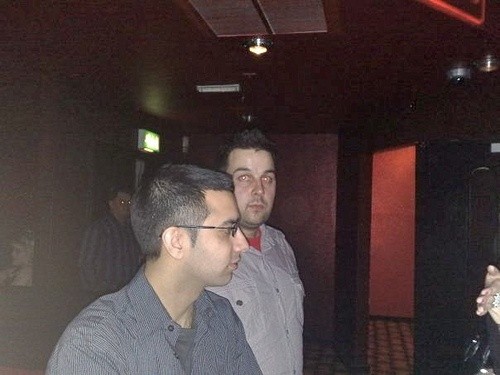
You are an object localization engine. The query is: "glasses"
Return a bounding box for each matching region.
[159,223,241,238]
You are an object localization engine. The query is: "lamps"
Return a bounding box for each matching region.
[241,38,274,56]
[479,55,499,72]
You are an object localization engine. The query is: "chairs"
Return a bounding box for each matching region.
[415,320,492,375]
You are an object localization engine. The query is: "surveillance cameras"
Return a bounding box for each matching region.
[445,68,474,86]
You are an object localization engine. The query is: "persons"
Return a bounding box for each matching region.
[0,231,36,288]
[475,227,500,375]
[78,181,146,313]
[45,164,263,375]
[203,129,305,375]
